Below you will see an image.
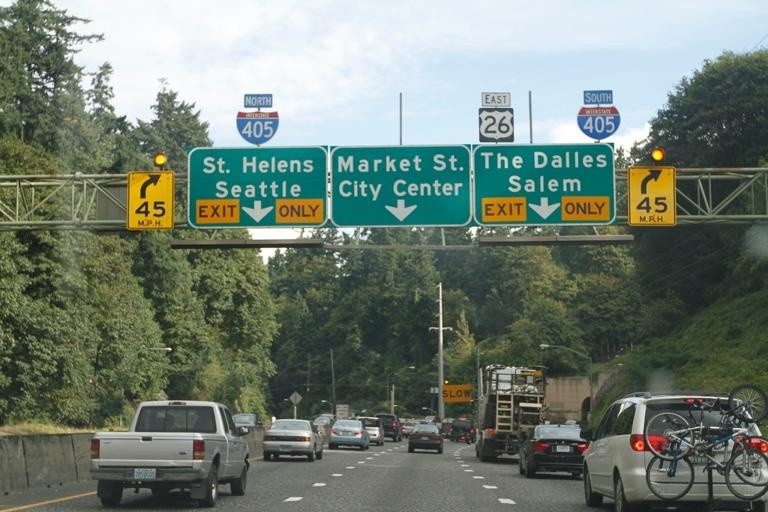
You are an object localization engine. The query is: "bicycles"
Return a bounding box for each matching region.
[644,382,767,504]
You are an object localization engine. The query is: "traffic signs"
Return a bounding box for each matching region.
[628,165,679,227]
[127,171,175,231]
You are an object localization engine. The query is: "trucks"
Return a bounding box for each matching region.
[473,363,548,463]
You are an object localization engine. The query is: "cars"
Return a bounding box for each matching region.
[312,412,476,454]
[261,418,324,461]
[231,412,267,435]
[516,420,589,480]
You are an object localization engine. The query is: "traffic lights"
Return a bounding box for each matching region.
[153,149,168,169]
[652,146,666,165]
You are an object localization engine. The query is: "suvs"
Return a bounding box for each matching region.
[583,389,768,511]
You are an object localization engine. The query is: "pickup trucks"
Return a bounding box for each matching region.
[88,400,255,506]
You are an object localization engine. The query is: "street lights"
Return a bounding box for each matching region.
[422,407,437,417]
[284,398,297,420]
[539,343,593,423]
[387,365,416,414]
[321,399,333,414]
[118,347,173,427]
